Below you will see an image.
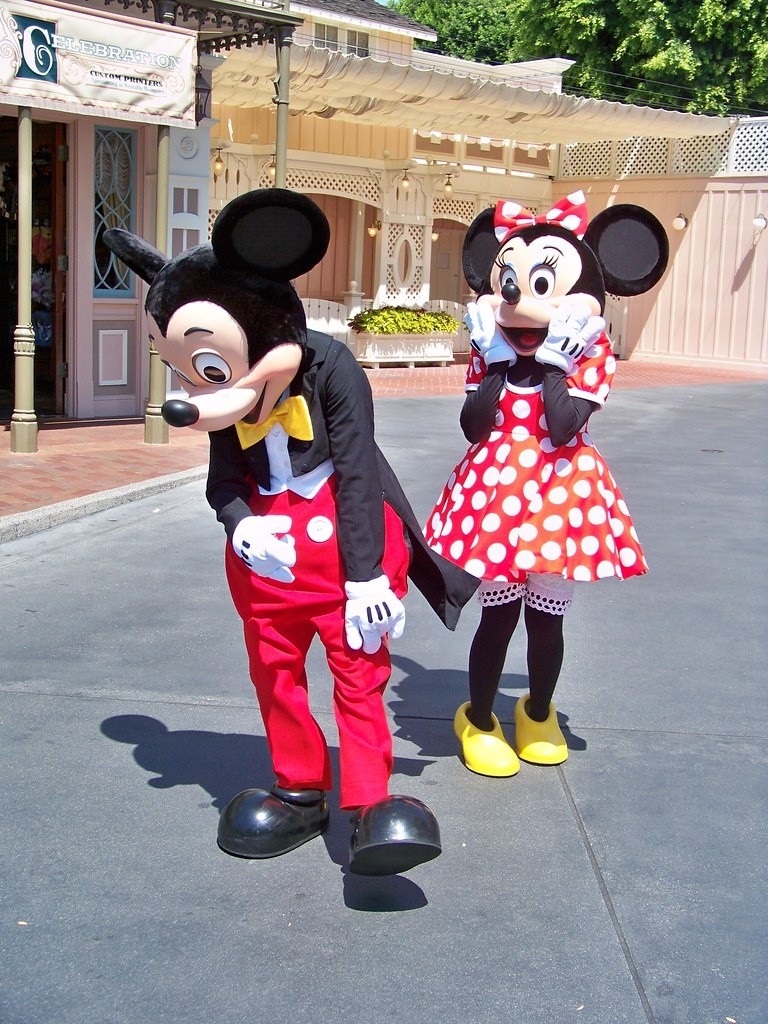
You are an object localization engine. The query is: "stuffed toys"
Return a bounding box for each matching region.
[423,189,670,777]
[104,187,482,876]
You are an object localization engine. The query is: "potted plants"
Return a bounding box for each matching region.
[346,303,463,371]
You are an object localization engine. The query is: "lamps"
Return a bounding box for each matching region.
[195,23,213,128]
[269,154,276,182]
[444,174,454,202]
[673,213,689,231]
[432,225,440,243]
[402,169,410,193]
[752,213,768,231]
[213,147,224,177]
[367,219,382,238]
